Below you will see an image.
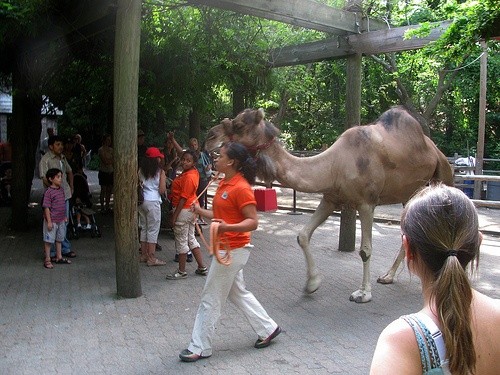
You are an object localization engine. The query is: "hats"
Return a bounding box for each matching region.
[146,147,164,159]
[138,130,145,135]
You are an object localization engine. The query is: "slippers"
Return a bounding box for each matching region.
[40,256,57,261]
[55,258,71,264]
[44,261,53,269]
[64,252,76,257]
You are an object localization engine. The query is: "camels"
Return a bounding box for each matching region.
[205,104,454,303]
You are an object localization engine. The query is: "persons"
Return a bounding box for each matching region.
[73,197,92,231]
[138,147,166,266]
[38,135,77,261]
[370,183,500,375]
[163,138,180,189]
[178,143,282,362]
[40,127,86,171]
[138,130,174,170]
[0,119,15,208]
[98,135,114,215]
[167,148,208,279]
[168,131,212,222]
[42,168,72,269]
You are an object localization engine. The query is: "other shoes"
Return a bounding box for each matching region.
[166,270,188,279]
[175,253,180,261]
[87,224,91,228]
[137,217,206,249]
[187,254,193,262]
[254,327,281,348]
[196,266,208,276]
[179,350,210,361]
[140,256,166,265]
[78,223,81,228]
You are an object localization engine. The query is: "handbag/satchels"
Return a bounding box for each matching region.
[138,180,144,206]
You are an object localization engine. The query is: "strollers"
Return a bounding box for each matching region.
[67,172,102,240]
